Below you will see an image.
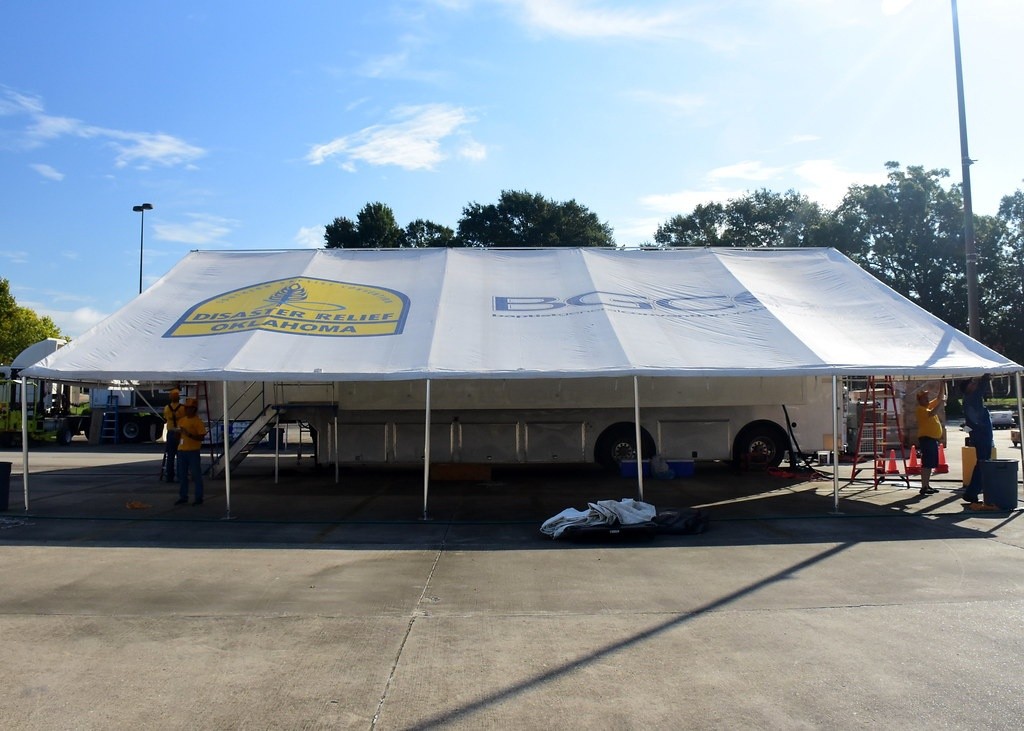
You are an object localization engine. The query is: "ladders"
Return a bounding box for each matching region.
[97,395,120,444]
[849,375,911,491]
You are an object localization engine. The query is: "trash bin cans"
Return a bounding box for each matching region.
[981,457,1020,510]
[0,461,14,512]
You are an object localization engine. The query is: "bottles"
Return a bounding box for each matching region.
[632,448,636,461]
[991,440,994,447]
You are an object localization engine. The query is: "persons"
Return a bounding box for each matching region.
[164,390,184,484]
[962,373,993,503]
[174,398,206,506]
[916,389,945,495]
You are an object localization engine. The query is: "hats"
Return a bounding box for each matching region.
[169,389,179,400]
[916,389,929,399]
[183,397,198,407]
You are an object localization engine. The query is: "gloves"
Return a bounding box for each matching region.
[982,374,992,384]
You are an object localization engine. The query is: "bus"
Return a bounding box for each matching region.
[935,443,948,473]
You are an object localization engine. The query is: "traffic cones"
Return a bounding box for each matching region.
[906,445,921,474]
[886,449,899,473]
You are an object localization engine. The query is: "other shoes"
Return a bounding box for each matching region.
[963,494,984,504]
[929,486,939,493]
[174,497,188,505]
[192,499,203,506]
[919,486,934,494]
[166,470,175,479]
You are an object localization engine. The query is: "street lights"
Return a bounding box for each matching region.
[132,201,154,295]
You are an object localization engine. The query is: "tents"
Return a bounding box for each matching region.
[16,248,1024,520]
